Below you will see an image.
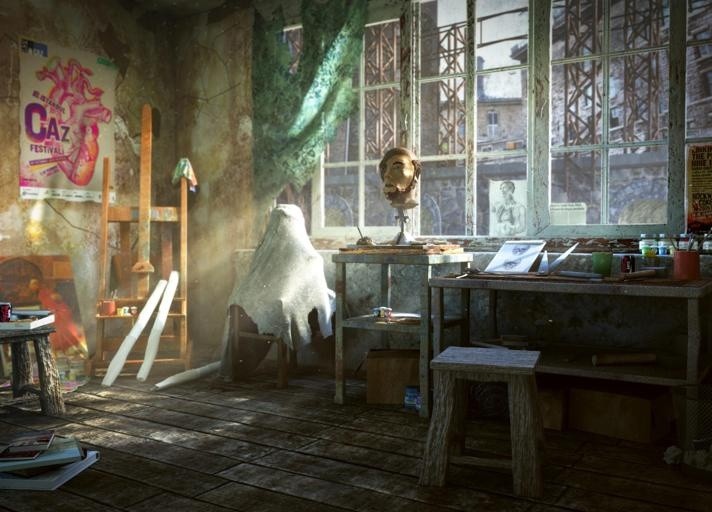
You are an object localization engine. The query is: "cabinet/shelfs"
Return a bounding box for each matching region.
[328,251,475,420]
[432,272,710,468]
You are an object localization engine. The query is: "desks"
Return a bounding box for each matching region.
[0,313,66,423]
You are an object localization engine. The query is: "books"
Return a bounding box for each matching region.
[0,429,100,493]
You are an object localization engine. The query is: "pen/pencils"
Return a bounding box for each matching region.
[356,225,364,242]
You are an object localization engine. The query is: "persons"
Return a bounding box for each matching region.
[489,181,526,235]
[375,147,421,209]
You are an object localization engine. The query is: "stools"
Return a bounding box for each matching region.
[420,345,551,498]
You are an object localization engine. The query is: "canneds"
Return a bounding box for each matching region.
[0,302,12,323]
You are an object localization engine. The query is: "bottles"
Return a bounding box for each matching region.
[116,306,138,315]
[404,386,423,410]
[637,233,659,255]
[656,234,677,256]
[678,234,699,253]
[702,234,712,254]
[372,306,392,319]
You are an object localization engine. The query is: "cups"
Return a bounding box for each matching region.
[591,251,613,277]
[102,300,115,316]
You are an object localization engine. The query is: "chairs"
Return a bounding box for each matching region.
[230,247,335,389]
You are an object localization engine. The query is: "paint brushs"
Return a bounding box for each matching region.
[668,227,712,251]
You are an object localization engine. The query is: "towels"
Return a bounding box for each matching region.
[170,156,200,194]
